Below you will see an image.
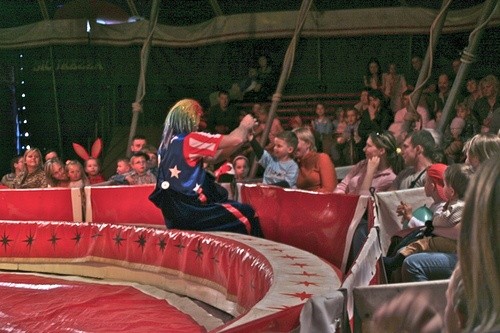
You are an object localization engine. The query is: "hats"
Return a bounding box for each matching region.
[426,163,449,201]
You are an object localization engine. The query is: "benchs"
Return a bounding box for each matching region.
[1,214,348,333]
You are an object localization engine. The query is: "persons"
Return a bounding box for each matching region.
[148,98,265,239]
[442,153,500,333]
[1,51,500,194]
[399,132,500,284]
[385,163,450,256]
[371,289,445,333]
[380,164,474,284]
